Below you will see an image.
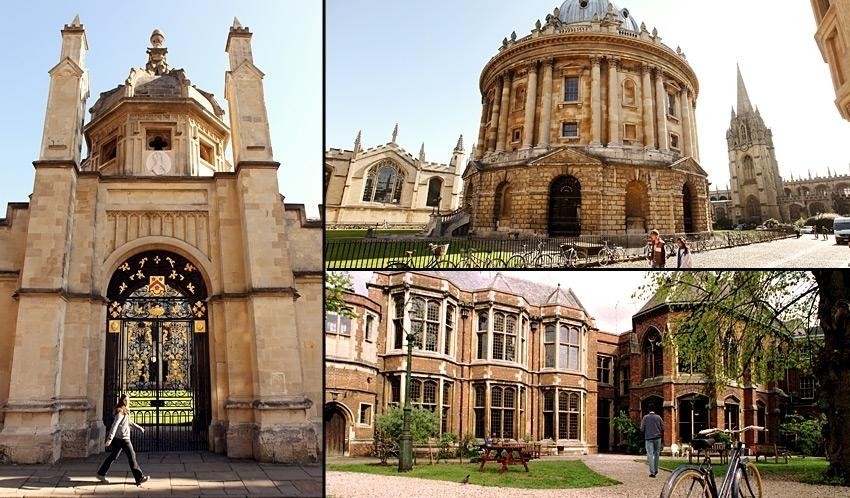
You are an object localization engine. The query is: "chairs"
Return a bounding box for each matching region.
[473,436,542,475]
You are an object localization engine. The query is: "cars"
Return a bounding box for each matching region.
[801,225,814,230]
[756,225,767,231]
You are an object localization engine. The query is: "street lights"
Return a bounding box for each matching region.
[397,288,425,468]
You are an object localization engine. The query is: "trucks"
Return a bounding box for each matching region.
[832,217,850,243]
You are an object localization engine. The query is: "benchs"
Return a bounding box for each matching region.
[661,446,672,456]
[687,439,791,465]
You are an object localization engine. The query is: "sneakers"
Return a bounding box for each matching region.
[137,475,150,485]
[95,473,109,485]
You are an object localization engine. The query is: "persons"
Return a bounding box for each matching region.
[677,236,693,269]
[821,225,829,241]
[647,229,667,268]
[638,402,666,478]
[793,223,801,239]
[813,225,819,239]
[96,394,152,487]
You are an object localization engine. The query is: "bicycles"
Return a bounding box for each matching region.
[659,423,769,498]
[386,239,588,269]
[644,229,790,261]
[595,239,626,266]
